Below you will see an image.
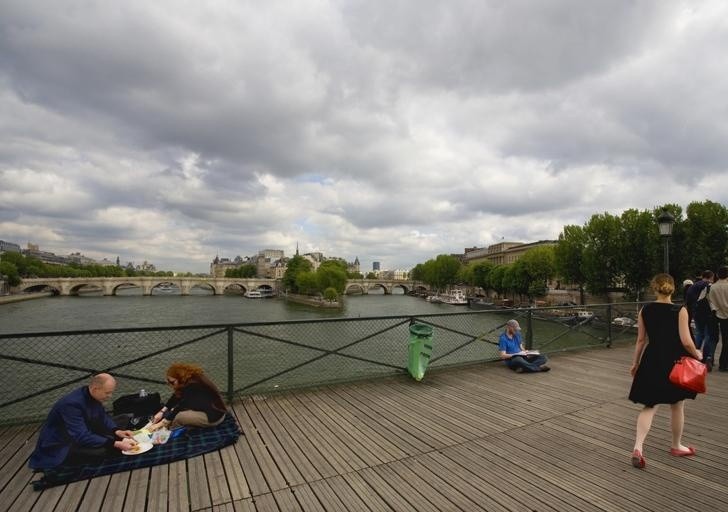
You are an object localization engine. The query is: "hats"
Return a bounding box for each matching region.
[506,319,521,331]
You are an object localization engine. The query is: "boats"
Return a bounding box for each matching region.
[433,289,468,305]
[257,289,275,297]
[407,290,427,298]
[160,283,173,293]
[244,289,262,299]
[469,293,640,327]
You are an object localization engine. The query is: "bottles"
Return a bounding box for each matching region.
[139,388,148,398]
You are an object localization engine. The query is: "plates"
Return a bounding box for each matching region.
[121,442,153,456]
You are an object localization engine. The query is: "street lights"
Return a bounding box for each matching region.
[657,206,676,275]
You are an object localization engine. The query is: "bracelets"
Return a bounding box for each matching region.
[159,409,164,414]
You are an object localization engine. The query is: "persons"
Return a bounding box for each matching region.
[682,266,728,373]
[499,320,551,374]
[30,373,132,471]
[630,273,703,469]
[148,363,227,432]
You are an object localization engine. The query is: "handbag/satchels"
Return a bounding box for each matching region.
[669,357,707,393]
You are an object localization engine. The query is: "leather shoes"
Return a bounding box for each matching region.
[632,450,645,468]
[516,366,550,373]
[671,446,695,457]
